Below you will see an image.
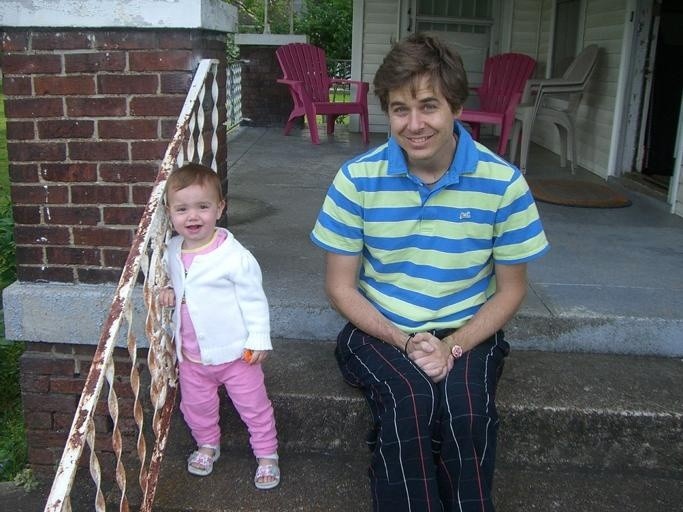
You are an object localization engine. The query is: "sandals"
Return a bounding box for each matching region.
[187,443,220,477]
[254,450,280,489]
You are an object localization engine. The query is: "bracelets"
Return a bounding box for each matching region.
[402,334,413,356]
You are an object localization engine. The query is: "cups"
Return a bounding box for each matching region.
[256,34,264,42]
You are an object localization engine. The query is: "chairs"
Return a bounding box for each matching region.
[274,41,372,147]
[457,43,602,178]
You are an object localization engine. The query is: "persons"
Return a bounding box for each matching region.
[157,163,282,491]
[306,34,554,512]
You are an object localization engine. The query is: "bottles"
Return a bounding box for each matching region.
[264,23,271,42]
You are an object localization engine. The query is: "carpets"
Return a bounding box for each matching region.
[529,179,633,209]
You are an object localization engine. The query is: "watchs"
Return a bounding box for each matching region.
[443,336,464,361]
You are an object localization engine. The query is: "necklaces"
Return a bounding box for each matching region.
[421,133,458,185]
